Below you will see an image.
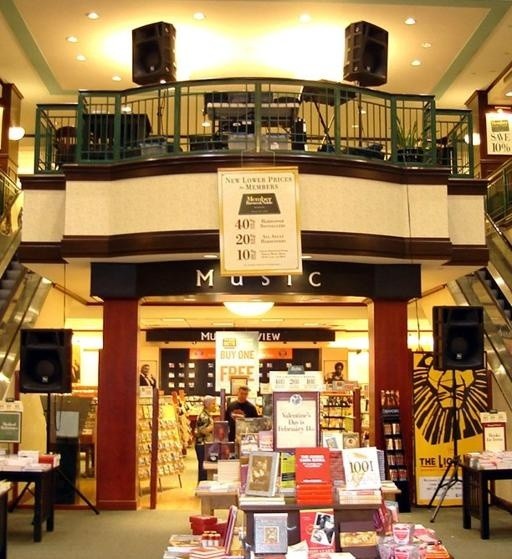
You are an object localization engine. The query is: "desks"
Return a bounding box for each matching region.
[450,456,511,539]
[1,486,11,558]
[0,462,62,542]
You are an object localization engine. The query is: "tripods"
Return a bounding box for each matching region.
[9,393,100,524]
[427,371,512,523]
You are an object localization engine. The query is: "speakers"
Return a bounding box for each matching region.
[132,21,177,85]
[18,329,71,393]
[344,20,388,86]
[433,306,484,371]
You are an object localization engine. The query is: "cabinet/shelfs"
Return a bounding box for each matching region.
[74,375,451,559]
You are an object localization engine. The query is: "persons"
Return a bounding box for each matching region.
[225,386,259,441]
[194,394,217,484]
[139,364,156,389]
[324,362,344,384]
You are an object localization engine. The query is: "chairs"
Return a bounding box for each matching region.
[54,125,78,170]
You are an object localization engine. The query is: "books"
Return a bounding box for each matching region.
[0,449,61,472]
[137,395,184,477]
[260,377,371,427]
[463,447,512,471]
[162,429,450,559]
[381,387,407,481]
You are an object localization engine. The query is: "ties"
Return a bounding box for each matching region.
[145,375,153,385]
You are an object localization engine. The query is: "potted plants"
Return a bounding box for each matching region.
[393,115,423,162]
